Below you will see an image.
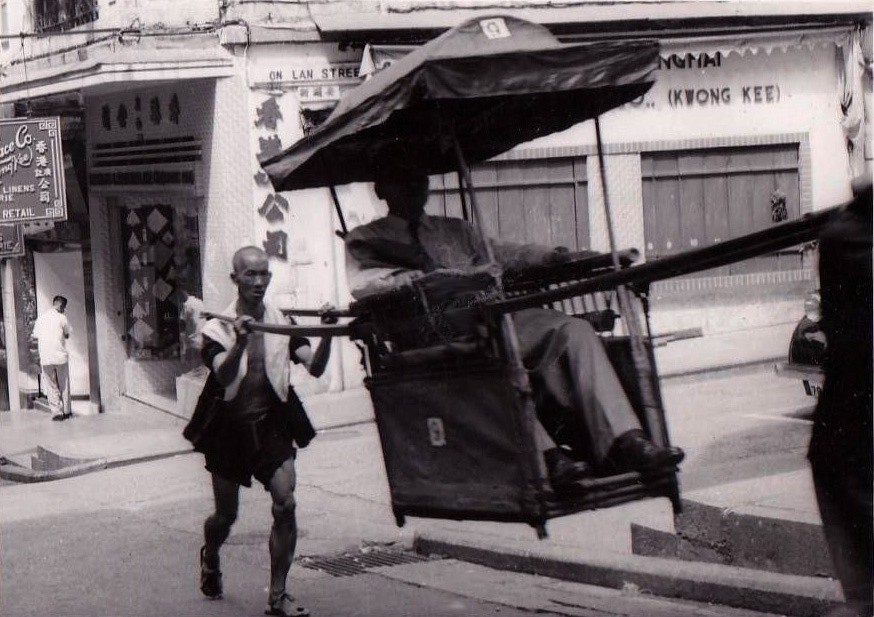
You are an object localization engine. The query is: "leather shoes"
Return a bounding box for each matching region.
[619,436,686,471]
[545,448,586,482]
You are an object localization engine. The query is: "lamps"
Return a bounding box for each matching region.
[78,92,87,110]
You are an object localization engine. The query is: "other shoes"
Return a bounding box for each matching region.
[52,415,64,422]
[65,413,72,419]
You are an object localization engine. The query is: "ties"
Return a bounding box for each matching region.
[407,221,432,261]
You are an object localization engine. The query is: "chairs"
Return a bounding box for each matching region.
[338,230,639,369]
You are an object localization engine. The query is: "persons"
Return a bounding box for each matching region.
[32,295,73,420]
[345,156,685,484]
[180,289,206,372]
[181,246,337,617]
[805,177,874,617]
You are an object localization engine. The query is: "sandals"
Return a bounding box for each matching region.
[266,592,308,616]
[200,547,222,598]
